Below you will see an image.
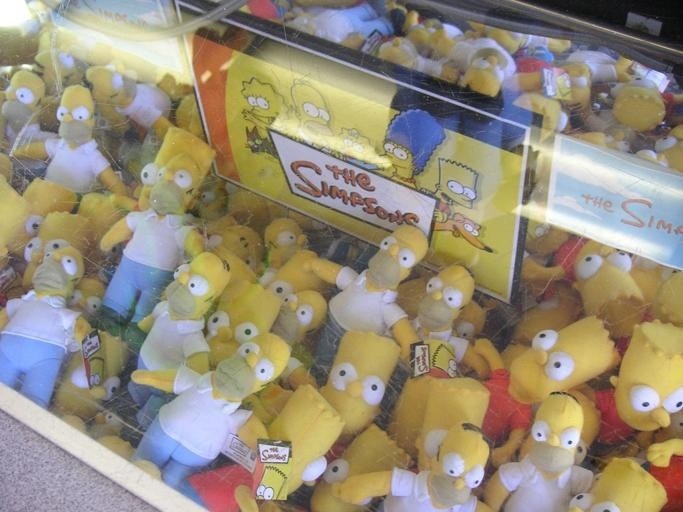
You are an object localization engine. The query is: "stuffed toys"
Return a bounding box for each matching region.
[1,0,682,512]
